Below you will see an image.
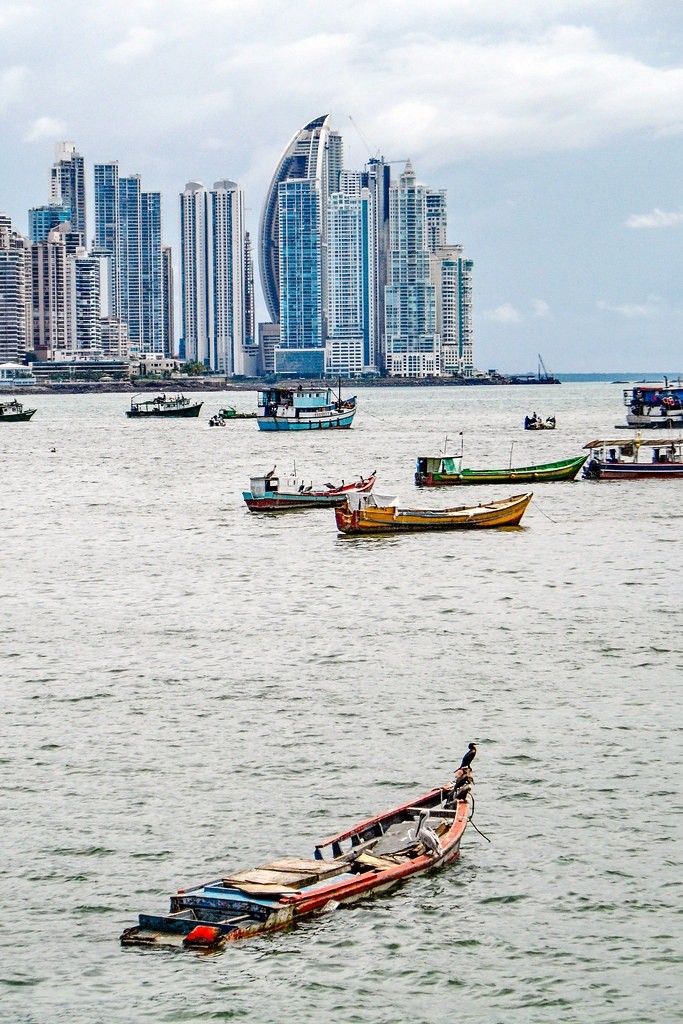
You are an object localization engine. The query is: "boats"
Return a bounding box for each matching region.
[124,392,205,418]
[523,411,557,429]
[625,376,683,429]
[241,460,377,513]
[414,431,591,487]
[257,372,357,431]
[581,431,683,479]
[219,406,258,419]
[333,490,534,535]
[117,741,476,954]
[0,399,37,421]
